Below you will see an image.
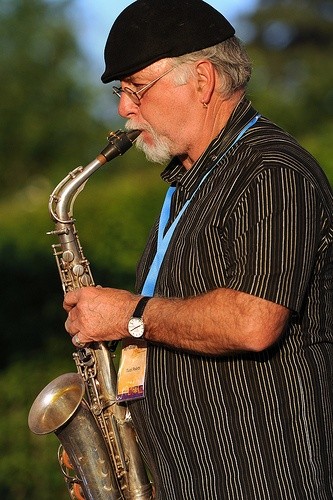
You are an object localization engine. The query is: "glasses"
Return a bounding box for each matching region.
[112,66,178,105]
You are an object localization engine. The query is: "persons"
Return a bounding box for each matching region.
[63,0,333,500]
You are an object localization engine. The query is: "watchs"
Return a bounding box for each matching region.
[128,296,154,341]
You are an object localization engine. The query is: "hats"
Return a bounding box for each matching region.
[100,0,235,84]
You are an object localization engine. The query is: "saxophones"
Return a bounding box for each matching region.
[28,128,154,499]
